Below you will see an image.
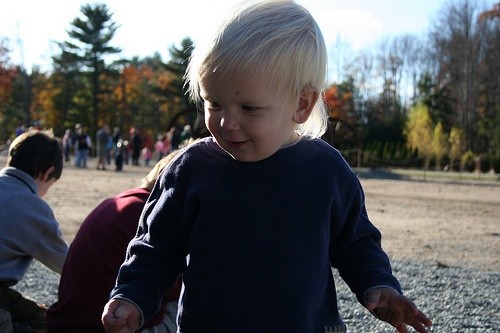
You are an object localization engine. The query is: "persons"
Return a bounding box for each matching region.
[50,143,186,333]
[6,121,210,172]
[102,0,433,333]
[0,131,69,333]
